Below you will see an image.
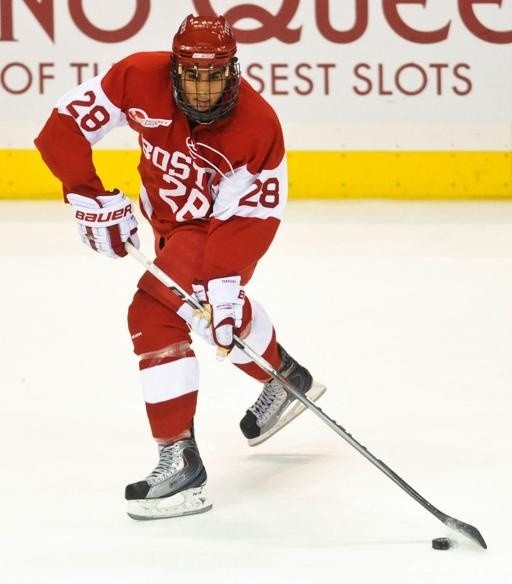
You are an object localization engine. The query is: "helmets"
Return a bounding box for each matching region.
[168,14,241,124]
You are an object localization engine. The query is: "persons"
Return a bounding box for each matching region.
[34,15,314,501]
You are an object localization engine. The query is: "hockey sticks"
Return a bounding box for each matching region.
[123,238,490,550]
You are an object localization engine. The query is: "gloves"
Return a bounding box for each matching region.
[190,273,246,359]
[66,187,140,259]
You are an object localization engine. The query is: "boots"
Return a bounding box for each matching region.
[239,342,313,439]
[125,417,207,500]
